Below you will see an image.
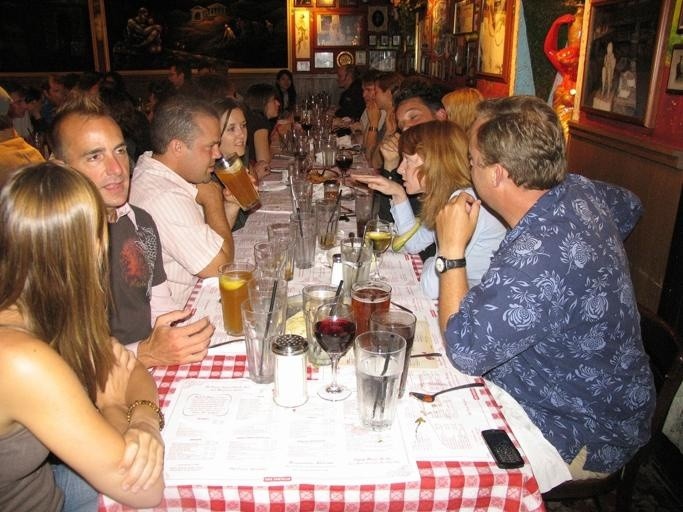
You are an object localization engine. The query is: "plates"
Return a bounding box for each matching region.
[314,149,336,170]
[309,168,340,184]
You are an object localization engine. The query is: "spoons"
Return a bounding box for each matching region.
[408,383,486,402]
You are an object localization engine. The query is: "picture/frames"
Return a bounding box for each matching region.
[579,0,683,129]
[453,0,514,83]
[100,0,293,76]
[0,0,100,78]
[294,0,368,73]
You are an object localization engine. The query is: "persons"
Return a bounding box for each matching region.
[330,63,656,487]
[0,58,299,512]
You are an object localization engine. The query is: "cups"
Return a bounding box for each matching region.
[294,181,313,217]
[241,295,289,386]
[370,308,416,400]
[315,199,341,249]
[290,212,318,269]
[213,152,262,215]
[218,262,257,338]
[339,237,374,303]
[300,110,313,135]
[301,284,346,368]
[268,221,294,285]
[353,331,407,433]
[323,180,340,200]
[255,240,288,280]
[351,279,393,353]
[248,277,288,318]
[296,141,317,175]
[354,192,380,236]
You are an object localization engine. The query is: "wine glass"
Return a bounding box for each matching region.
[313,304,358,401]
[294,90,393,401]
[144,105,151,116]
[335,150,353,187]
[363,219,392,281]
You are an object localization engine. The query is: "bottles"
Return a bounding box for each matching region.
[33,131,40,148]
[271,334,309,408]
[136,97,142,111]
[329,253,343,285]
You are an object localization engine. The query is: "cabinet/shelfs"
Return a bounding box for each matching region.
[565,121,683,313]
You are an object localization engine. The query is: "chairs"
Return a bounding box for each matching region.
[541,302,682,512]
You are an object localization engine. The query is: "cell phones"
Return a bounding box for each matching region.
[481,428,524,470]
